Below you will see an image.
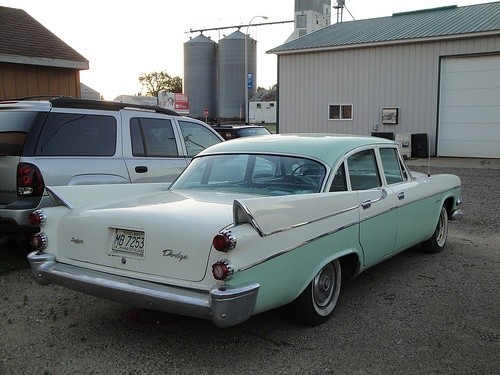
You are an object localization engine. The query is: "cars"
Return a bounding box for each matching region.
[209,122,272,141]
[27,132,464,329]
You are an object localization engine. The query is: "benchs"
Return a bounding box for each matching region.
[238,181,346,192]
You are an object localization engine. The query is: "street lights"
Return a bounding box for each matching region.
[244,16,269,125]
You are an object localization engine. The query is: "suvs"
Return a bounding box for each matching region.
[0,94,276,253]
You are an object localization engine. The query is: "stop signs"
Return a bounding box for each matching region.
[204,109,208,117]
